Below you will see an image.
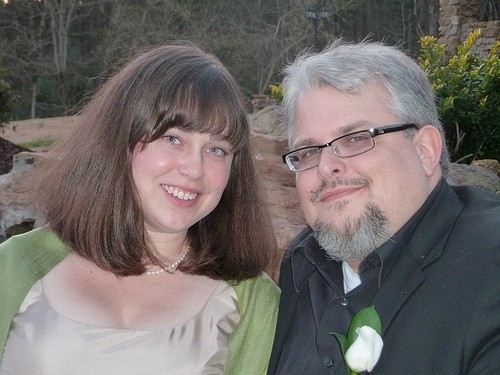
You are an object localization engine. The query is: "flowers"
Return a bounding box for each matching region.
[327,304,384,375]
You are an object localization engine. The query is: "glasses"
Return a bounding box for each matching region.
[282,123,418,172]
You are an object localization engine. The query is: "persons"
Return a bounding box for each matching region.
[0,45,281,375]
[267,43,500,375]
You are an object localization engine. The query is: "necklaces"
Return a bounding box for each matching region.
[145,249,190,275]
